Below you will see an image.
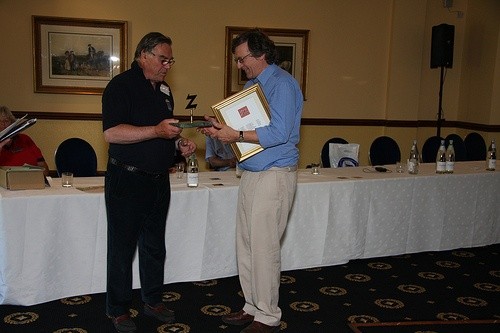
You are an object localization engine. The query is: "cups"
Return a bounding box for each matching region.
[312,163,320,175]
[61,172,74,187]
[396,161,405,173]
[175,162,184,180]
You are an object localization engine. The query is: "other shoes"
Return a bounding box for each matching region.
[111,314,137,332]
[221,309,256,328]
[240,320,282,333]
[142,302,176,321]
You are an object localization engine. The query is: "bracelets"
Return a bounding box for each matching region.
[178,140,181,149]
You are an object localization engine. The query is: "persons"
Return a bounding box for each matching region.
[170,125,241,172]
[102,31,197,332]
[198,29,304,333]
[0,106,49,179]
[64,43,97,71]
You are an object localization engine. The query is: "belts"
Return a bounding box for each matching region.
[108,158,141,172]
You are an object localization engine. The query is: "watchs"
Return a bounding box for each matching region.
[239,131,245,142]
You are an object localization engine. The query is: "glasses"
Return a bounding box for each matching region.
[234,52,252,65]
[149,52,175,67]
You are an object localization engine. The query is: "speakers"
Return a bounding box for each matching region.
[431,23,455,68]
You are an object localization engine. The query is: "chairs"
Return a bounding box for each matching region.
[55,138,97,177]
[321,132,487,168]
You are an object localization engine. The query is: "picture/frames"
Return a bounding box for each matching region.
[224,26,310,101]
[210,83,271,162]
[31,15,128,95]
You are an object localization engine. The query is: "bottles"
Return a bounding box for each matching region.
[485,138,497,171]
[436,140,447,174]
[407,144,420,174]
[186,153,199,187]
[446,140,455,174]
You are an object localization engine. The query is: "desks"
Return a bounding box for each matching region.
[0,161,500,306]
